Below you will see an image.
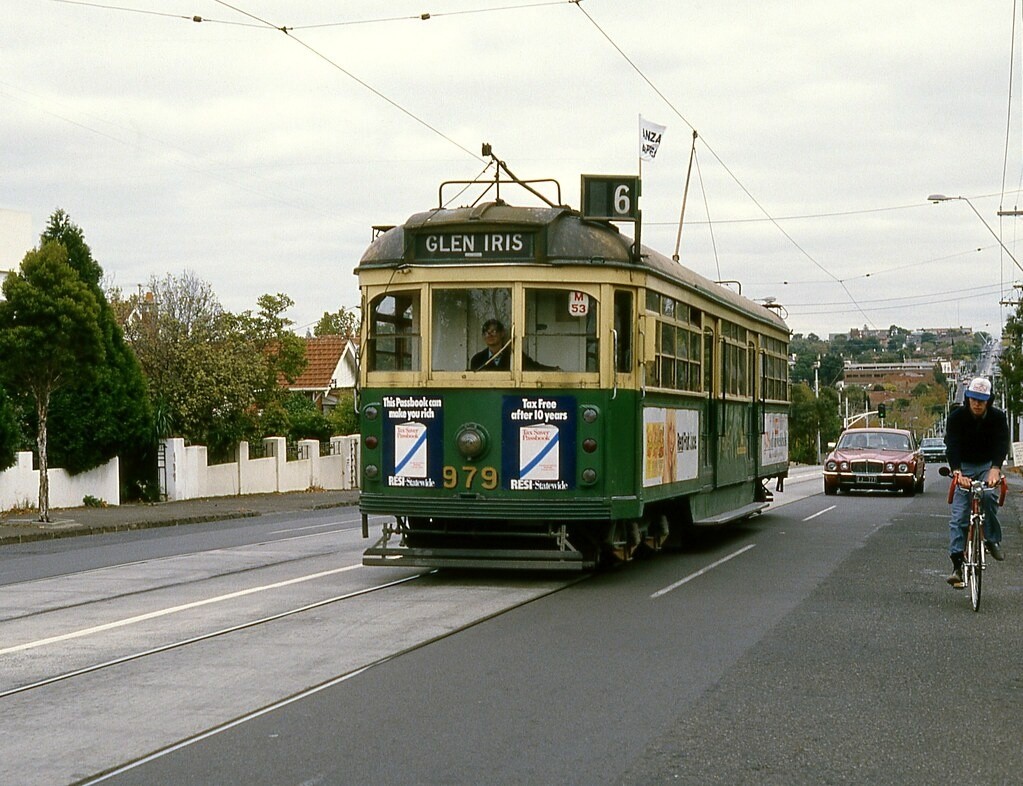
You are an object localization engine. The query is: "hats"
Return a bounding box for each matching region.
[965,378,991,400]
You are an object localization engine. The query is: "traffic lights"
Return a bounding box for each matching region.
[878,404,886,418]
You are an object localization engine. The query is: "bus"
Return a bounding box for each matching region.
[354,200,792,571]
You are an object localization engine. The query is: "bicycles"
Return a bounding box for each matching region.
[939,466,1006,611]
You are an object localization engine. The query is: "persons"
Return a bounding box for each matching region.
[856,435,867,447]
[661,409,676,484]
[470,319,562,372]
[947,378,1011,591]
[926,441,932,445]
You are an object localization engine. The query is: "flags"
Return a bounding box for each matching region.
[639,116,666,163]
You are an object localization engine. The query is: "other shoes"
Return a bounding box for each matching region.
[985,540,1004,560]
[946,569,962,586]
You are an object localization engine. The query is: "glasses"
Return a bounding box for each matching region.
[484,329,501,338]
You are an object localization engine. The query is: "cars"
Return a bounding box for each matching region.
[918,437,948,463]
[822,428,926,497]
[959,373,974,385]
